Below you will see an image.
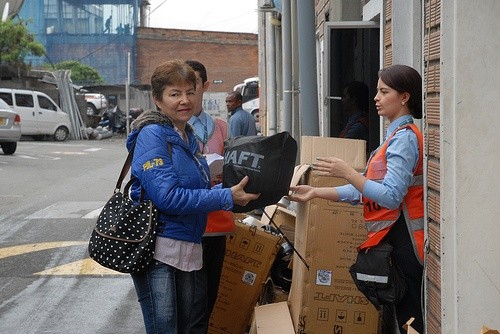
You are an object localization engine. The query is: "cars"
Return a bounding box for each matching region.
[81,92,108,117]
[0,97,21,155]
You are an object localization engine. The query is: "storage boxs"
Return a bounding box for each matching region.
[206,133,389,334]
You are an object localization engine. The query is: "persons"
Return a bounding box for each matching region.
[284,64,424,334]
[225,91,258,137]
[335,81,370,153]
[125,60,260,334]
[176,60,235,333]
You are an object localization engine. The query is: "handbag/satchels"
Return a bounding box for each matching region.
[351,241,408,311]
[222,131,298,213]
[87,176,159,274]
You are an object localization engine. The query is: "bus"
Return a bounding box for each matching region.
[233,76,261,123]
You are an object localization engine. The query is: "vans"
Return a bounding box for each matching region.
[0,87,72,141]
